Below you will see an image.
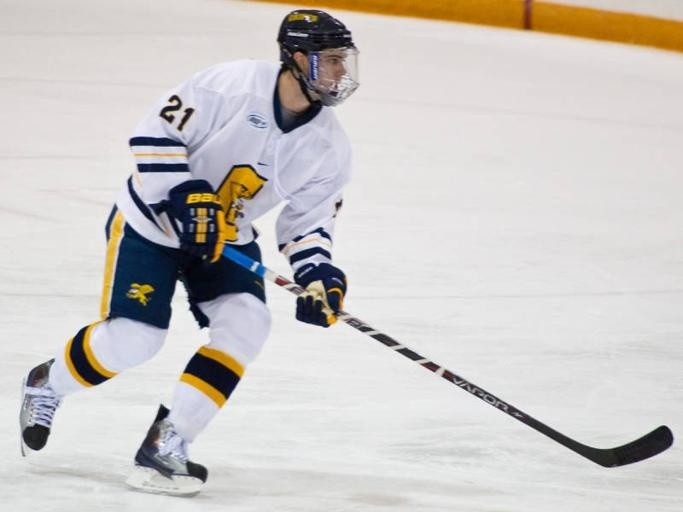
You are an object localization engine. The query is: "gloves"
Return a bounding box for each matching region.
[292,261,347,329]
[166,178,239,266]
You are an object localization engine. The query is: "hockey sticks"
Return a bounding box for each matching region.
[224,245,673,468]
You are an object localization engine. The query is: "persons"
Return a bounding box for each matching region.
[17,7,362,487]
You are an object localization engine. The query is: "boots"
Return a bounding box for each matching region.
[17,358,61,452]
[132,403,209,485]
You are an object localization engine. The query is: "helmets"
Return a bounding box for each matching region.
[274,7,362,108]
